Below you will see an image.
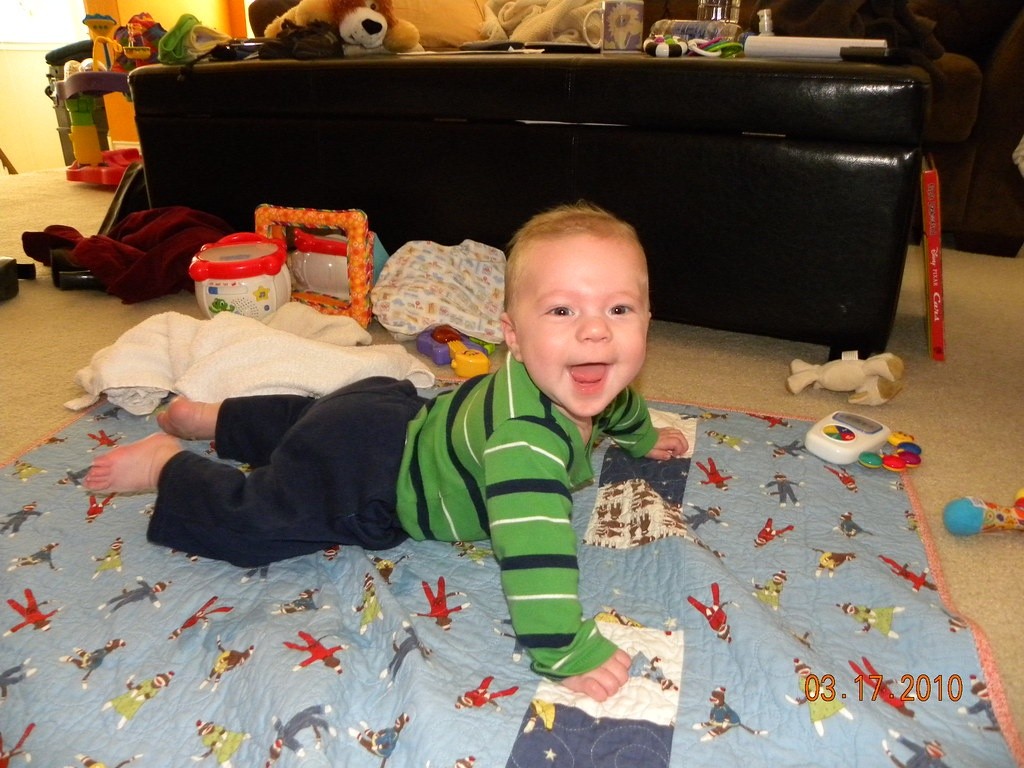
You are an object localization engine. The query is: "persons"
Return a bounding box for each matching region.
[81,204,689,704]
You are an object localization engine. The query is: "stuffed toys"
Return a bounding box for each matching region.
[264,0,419,55]
[785,351,906,406]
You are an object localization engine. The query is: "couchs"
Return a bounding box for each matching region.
[127,47,929,362]
[249,0,1024,258]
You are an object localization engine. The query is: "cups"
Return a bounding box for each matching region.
[582,0,645,55]
[696,0,740,24]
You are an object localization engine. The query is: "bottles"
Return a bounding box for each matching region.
[649,19,755,51]
